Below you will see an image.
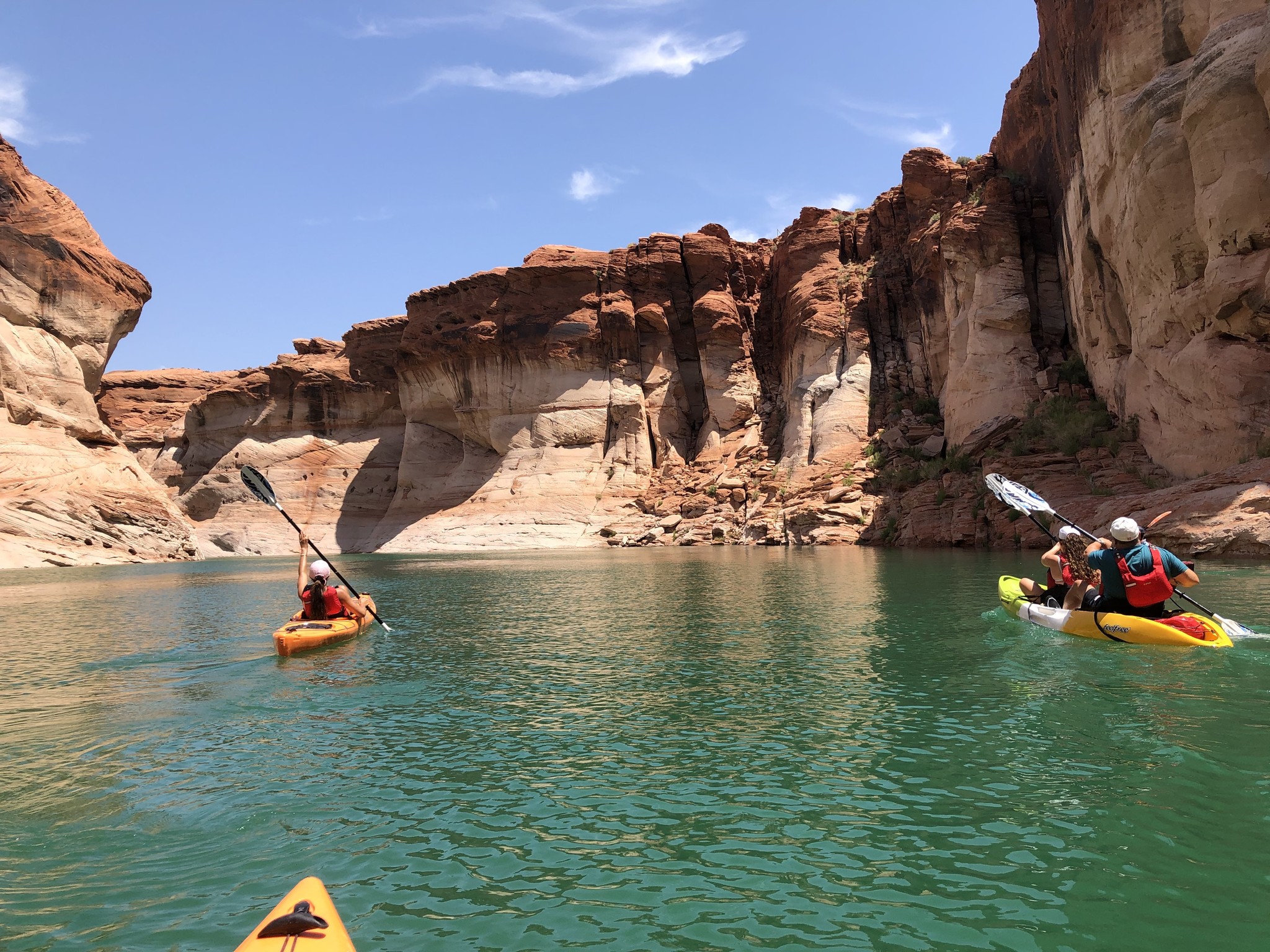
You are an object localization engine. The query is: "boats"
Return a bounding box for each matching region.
[997,574,1235,649]
[272,592,377,657]
[228,874,358,952]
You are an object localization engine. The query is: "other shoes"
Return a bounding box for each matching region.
[1045,596,1061,609]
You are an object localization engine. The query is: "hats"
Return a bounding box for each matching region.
[309,560,330,580]
[1110,516,1139,541]
[1058,525,1081,540]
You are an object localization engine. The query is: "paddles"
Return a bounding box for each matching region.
[985,472,1059,542]
[1002,480,1257,636]
[241,465,395,634]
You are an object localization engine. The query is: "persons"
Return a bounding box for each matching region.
[297,530,369,620]
[1019,526,1102,604]
[1045,517,1199,621]
